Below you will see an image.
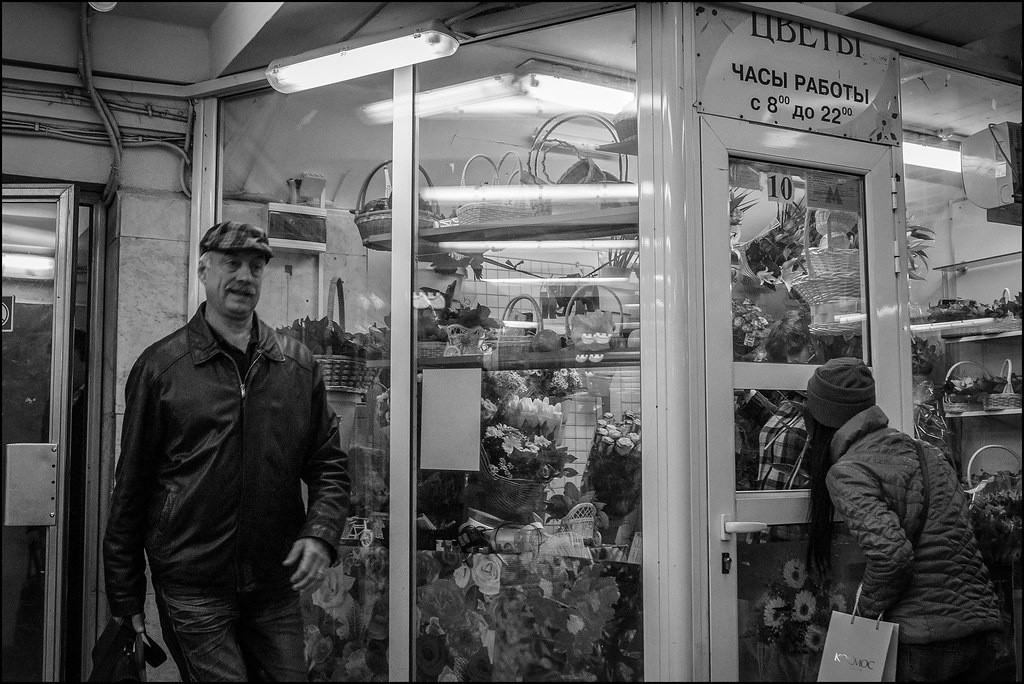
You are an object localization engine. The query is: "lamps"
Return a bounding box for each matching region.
[513,58,961,173]
[265,19,461,94]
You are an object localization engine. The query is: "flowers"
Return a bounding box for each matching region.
[272,191,1024,684]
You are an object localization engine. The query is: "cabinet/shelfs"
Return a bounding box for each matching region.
[945,330,1022,418]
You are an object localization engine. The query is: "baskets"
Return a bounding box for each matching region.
[941,288,1022,414]
[457,152,533,224]
[439,324,483,357]
[482,294,544,352]
[615,118,637,143]
[783,209,861,336]
[418,341,447,358]
[310,277,384,394]
[354,158,442,238]
[565,284,627,349]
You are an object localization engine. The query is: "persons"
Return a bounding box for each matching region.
[102,223,352,684]
[26,328,89,684]
[803,357,1005,683]
[757,311,820,490]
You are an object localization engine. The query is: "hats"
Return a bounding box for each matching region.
[807,356,877,429]
[198,222,274,265]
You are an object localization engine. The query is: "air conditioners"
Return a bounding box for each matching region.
[961,121,1024,228]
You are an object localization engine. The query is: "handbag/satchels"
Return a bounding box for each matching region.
[816,583,900,683]
[86,617,168,684]
